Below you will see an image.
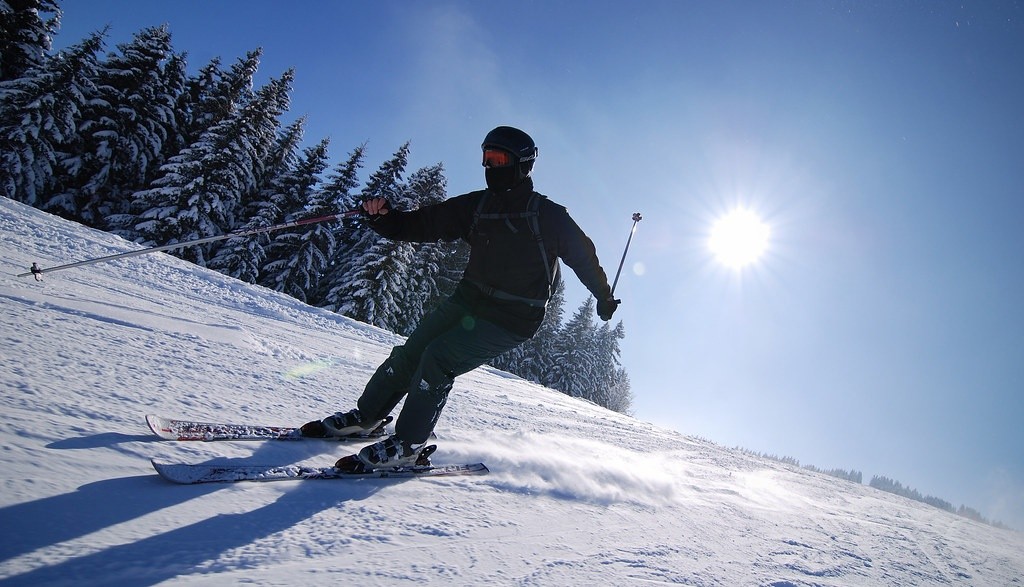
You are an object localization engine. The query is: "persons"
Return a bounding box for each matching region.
[323,125,618,473]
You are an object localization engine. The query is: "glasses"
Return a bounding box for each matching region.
[482,149,520,169]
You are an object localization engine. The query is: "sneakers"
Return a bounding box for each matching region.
[321,408,385,436]
[358,430,427,468]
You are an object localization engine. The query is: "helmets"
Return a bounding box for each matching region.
[481,126,538,189]
